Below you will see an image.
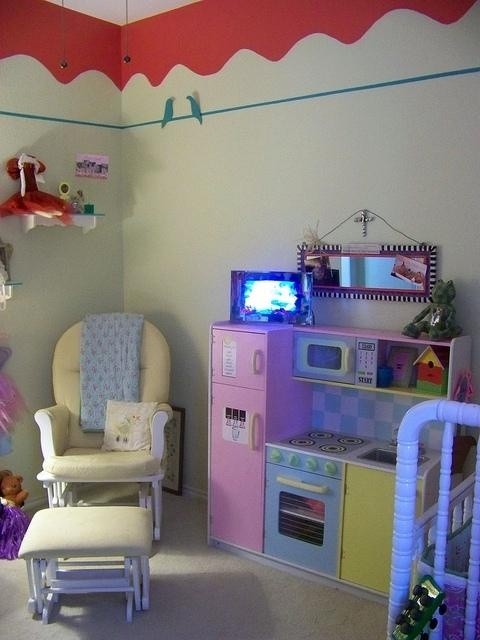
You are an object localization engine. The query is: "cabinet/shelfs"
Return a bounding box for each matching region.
[294,322,473,401]
[206,323,311,559]
[339,464,423,607]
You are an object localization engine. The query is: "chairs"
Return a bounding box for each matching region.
[30,314,171,540]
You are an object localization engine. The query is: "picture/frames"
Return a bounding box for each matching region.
[162,403,186,495]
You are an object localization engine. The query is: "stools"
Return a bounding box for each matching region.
[20,503,156,624]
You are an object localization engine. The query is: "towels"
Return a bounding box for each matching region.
[78,315,144,435]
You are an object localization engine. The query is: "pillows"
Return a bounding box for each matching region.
[100,397,157,453]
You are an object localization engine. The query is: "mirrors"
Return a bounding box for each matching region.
[289,244,437,305]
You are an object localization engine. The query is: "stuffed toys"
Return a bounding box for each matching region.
[0,469,29,508]
[402,278,464,341]
